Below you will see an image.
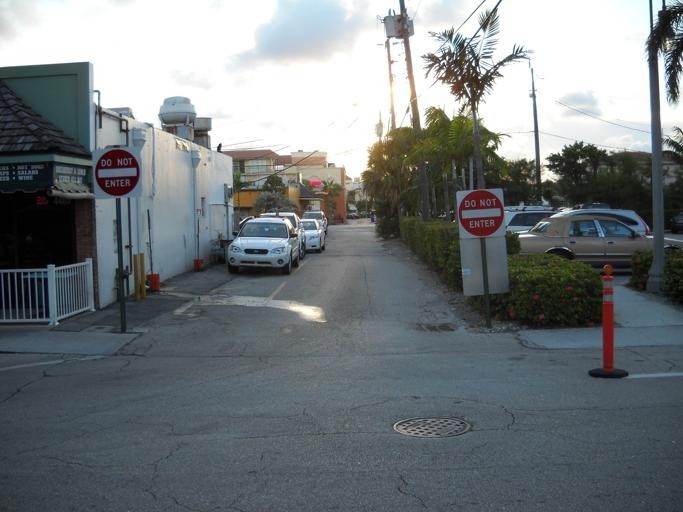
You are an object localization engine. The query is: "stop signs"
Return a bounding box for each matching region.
[92,147,140,198]
[455,189,504,240]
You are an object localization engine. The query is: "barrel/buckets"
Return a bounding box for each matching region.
[194,259,203,272]
[146,274,160,292]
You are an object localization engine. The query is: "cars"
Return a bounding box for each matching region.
[504,202,683,269]
[226,209,328,275]
